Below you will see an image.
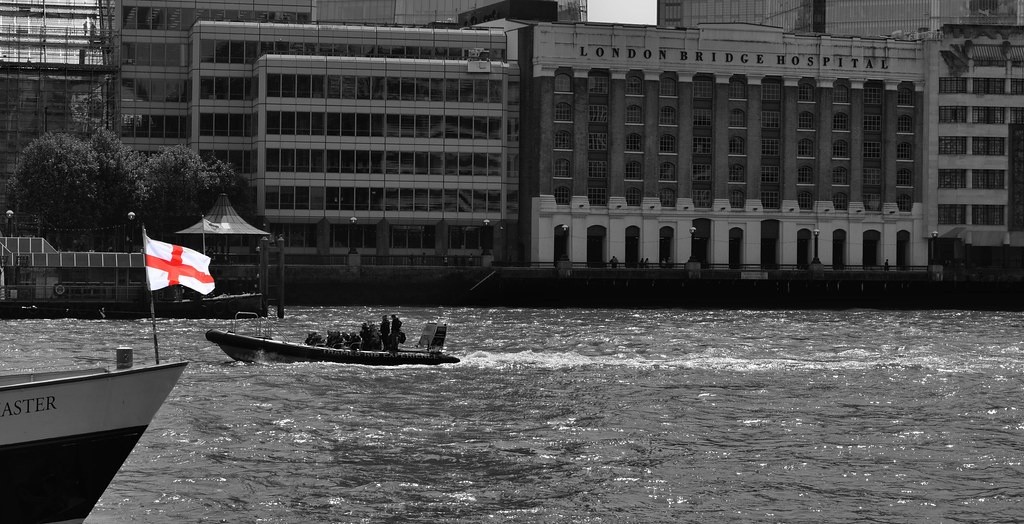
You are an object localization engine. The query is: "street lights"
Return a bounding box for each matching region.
[5,210,14,237]
[560,225,570,260]
[347,217,359,254]
[126,213,137,253]
[689,227,698,261]
[931,230,941,264]
[481,218,490,255]
[812,229,821,264]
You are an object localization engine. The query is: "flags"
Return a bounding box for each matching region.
[145,236,215,295]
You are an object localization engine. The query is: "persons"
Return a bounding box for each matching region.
[304,322,381,351]
[884,259,889,270]
[609,256,673,267]
[390,314,402,352]
[380,314,389,351]
[205,246,231,264]
[408,251,475,264]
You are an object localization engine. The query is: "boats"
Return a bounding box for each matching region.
[1,237,268,319]
[0,228,216,522]
[205,309,462,369]
